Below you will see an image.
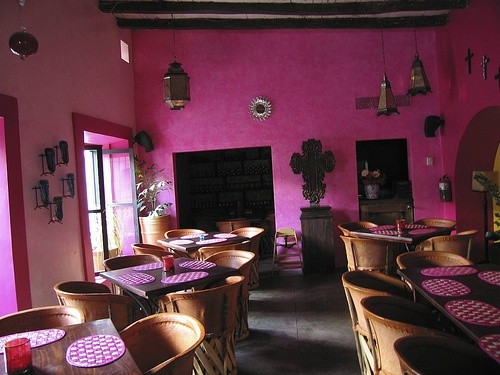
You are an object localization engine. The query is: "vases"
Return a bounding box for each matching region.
[364,184,379,199]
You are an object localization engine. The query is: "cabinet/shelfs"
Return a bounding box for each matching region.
[189,158,274,209]
[216,220,250,233]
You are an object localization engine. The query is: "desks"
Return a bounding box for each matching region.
[0,318,143,375]
[166,232,245,251]
[300,207,335,274]
[356,224,454,237]
[100,257,239,316]
[397,263,500,365]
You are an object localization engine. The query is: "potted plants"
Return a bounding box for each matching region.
[133,155,172,245]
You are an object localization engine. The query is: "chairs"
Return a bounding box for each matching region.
[338,218,500,375]
[0,227,264,375]
[272,227,304,275]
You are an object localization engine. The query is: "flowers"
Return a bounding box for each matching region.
[361,170,387,185]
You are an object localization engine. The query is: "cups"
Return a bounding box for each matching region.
[162,255,175,271]
[4,337,31,375]
[397,219,406,230]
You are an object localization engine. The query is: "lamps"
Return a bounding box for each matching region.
[406,28,432,97]
[163,13,190,110]
[377,29,400,117]
